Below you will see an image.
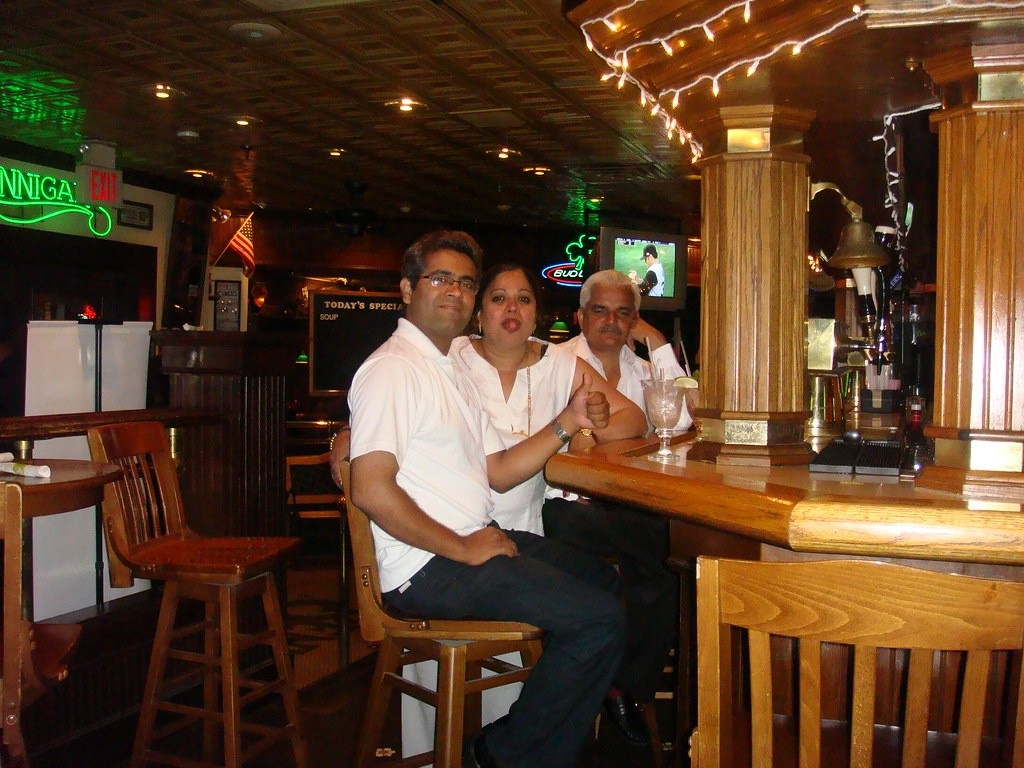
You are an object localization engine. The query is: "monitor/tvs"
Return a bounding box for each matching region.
[589,226,689,312]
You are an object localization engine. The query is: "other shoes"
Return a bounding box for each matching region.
[471,725,495,768]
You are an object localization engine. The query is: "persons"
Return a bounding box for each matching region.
[629,245,665,296]
[543,269,693,746]
[347,230,631,768]
[449,261,648,536]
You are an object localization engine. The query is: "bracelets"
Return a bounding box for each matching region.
[551,418,570,442]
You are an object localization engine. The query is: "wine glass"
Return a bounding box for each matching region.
[680,377,703,445]
[641,378,684,461]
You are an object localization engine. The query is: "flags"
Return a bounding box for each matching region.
[229,219,255,279]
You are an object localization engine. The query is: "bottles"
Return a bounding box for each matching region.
[904,403,925,470]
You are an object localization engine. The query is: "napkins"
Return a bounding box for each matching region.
[0,462,51,478]
[0,452,14,463]
[182,323,202,331]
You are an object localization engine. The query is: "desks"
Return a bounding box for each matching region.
[0,459,123,768]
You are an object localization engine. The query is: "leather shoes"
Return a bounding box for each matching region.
[602,691,653,747]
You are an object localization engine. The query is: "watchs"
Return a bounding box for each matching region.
[579,428,598,444]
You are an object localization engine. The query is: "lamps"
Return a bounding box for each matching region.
[806,176,890,269]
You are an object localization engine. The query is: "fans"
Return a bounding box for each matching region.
[247,180,466,244]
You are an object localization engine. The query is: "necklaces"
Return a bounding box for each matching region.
[513,340,531,438]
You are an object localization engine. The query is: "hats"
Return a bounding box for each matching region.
[640,245,657,260]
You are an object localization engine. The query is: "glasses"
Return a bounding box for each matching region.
[408,274,480,294]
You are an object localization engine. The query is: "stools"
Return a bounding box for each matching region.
[88,421,310,767]
[331,427,545,768]
[687,555,1024,768]
